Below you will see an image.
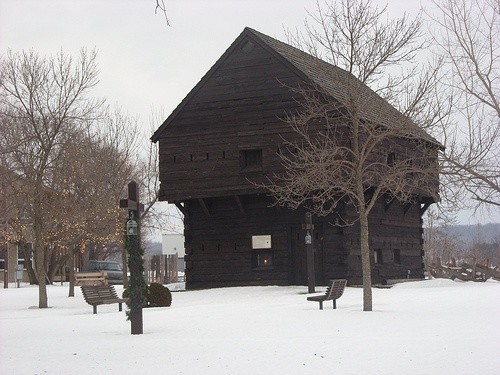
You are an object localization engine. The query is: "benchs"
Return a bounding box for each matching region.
[74,270,108,286]
[307,279,348,310]
[79,284,124,314]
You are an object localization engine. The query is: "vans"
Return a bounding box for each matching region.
[87,260,124,285]
